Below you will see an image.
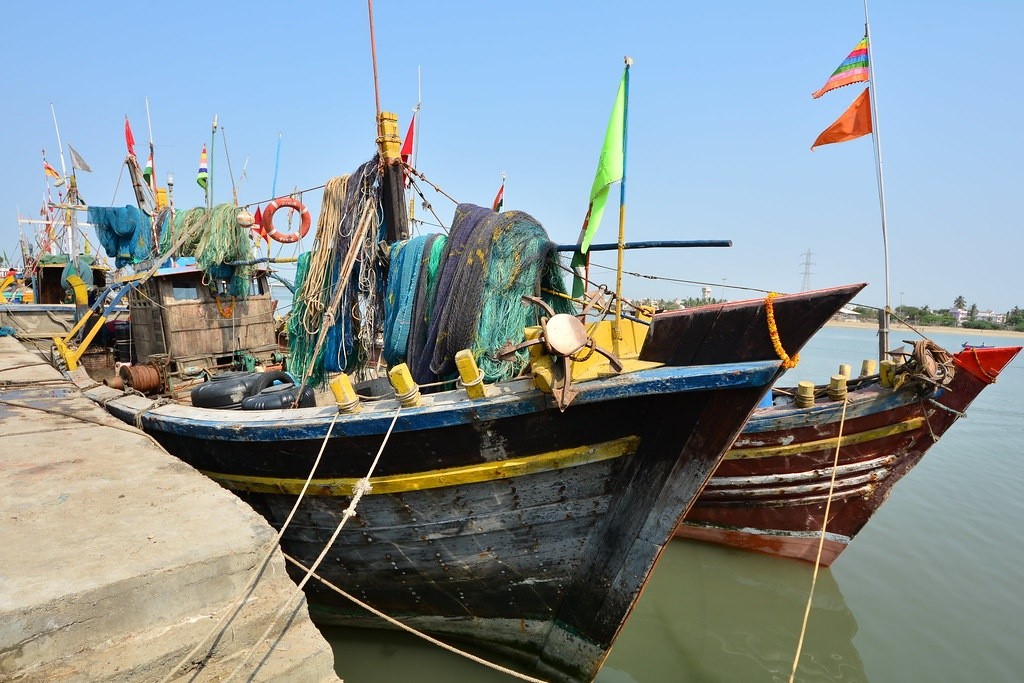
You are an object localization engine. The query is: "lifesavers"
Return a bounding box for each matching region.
[263,198,312,244]
[252,371,297,395]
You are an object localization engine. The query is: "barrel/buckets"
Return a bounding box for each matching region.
[114,322,133,361]
[81,345,118,381]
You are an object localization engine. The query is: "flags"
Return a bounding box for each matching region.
[69,147,92,173]
[494,186,503,211]
[126,122,135,155]
[45,163,60,179]
[143,155,152,186]
[570,69,625,302]
[198,147,208,188]
[401,116,414,181]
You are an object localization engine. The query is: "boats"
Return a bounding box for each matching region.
[1,0,870,683]
[665,6,1021,572]
[0,102,130,349]
